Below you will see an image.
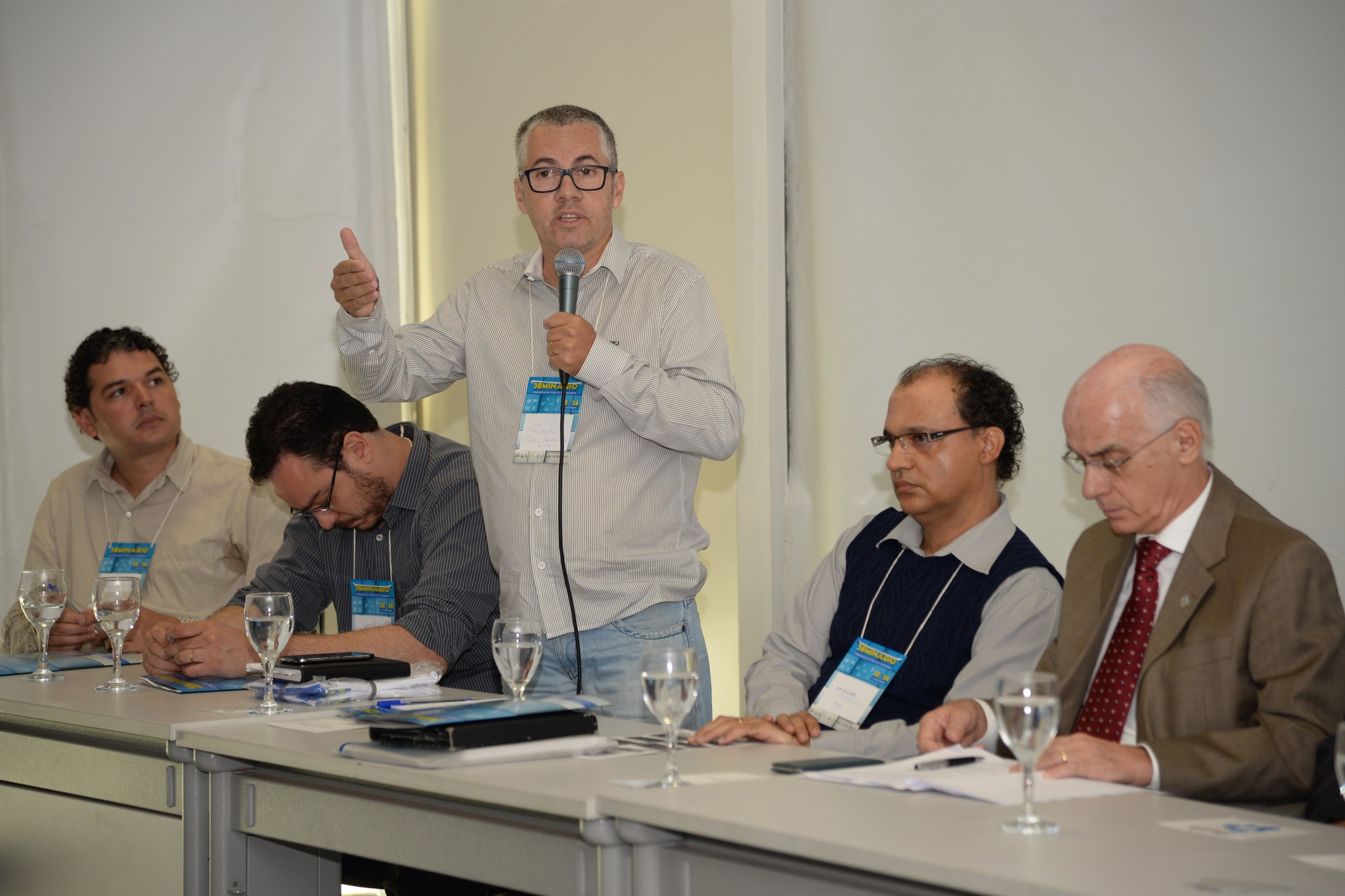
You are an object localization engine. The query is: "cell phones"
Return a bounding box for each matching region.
[280,651,374,665]
[772,757,885,776]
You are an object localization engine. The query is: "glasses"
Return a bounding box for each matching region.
[1062,426,1173,478]
[870,425,982,456]
[519,165,617,193]
[288,450,341,517]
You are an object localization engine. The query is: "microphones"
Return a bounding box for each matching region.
[553,248,586,386]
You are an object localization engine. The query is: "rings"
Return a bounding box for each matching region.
[737,717,744,724]
[189,648,194,662]
[549,340,556,355]
[1061,750,1068,762]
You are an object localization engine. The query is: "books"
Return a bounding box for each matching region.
[0,648,144,677]
[809,736,1157,805]
[136,672,253,693]
[321,680,621,768]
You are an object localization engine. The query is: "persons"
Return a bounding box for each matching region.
[137,379,509,695]
[916,343,1344,830]
[680,353,1065,758]
[2,324,291,661]
[323,93,740,733]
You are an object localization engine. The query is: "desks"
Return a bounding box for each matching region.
[0,643,1345,896]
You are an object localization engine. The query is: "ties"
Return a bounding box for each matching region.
[1069,536,1172,742]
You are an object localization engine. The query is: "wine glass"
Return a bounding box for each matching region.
[491,618,545,704]
[92,576,140,693]
[637,647,696,791]
[19,570,67,682]
[989,670,1062,835]
[243,592,296,715]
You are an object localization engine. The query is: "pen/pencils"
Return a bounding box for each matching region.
[914,755,985,770]
[170,634,176,645]
[376,698,473,706]
[48,582,103,635]
